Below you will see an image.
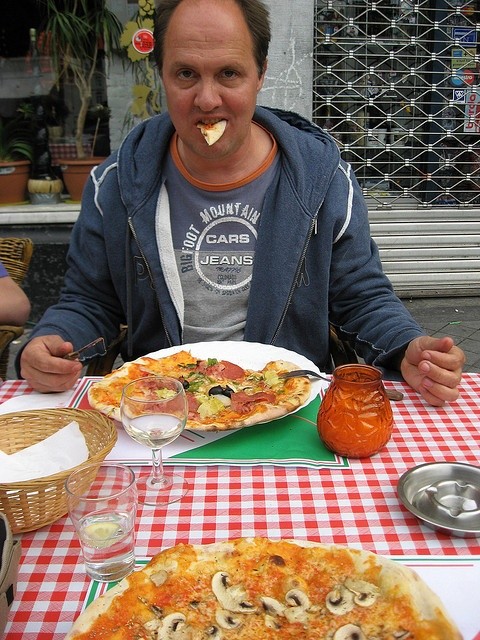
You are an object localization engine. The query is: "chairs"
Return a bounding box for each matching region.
[0,237,34,360]
[84,324,360,374]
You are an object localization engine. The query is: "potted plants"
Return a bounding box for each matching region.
[47,0,123,200]
[0,114,33,204]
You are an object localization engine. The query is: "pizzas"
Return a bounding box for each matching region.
[198,121,226,146]
[85,348,312,432]
[58,539,459,639]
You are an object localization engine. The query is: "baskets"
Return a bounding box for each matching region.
[0,407,118,535]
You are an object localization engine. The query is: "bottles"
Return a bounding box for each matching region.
[318,365,395,459]
[47,106,64,139]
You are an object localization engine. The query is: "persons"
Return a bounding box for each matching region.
[0,261,31,326]
[13,1,466,408]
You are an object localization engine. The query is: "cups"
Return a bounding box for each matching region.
[66,463,137,582]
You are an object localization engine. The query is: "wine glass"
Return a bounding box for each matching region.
[121,379,189,505]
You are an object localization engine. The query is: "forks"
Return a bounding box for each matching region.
[278,370,404,401]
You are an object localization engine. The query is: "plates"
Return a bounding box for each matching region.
[109,341,323,429]
